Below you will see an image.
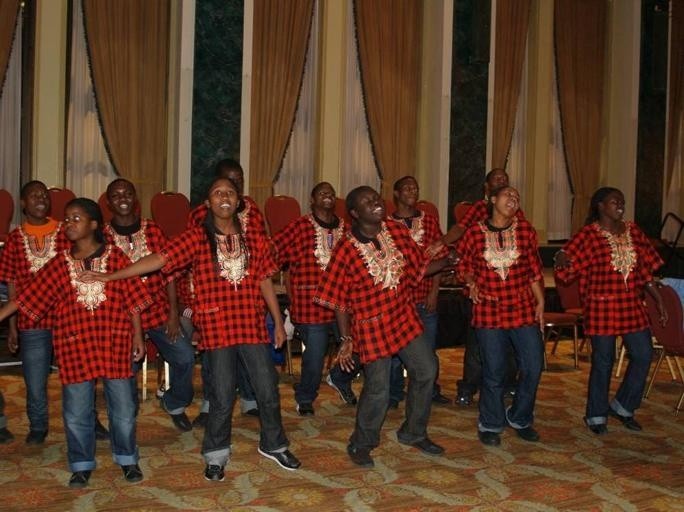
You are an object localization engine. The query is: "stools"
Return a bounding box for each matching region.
[542,310,579,370]
[140,331,294,400]
[614,331,684,384]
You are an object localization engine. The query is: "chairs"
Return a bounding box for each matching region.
[552,266,593,362]
[644,281,684,416]
[335,196,474,225]
[48,187,76,222]
[265,196,304,288]
[0,189,14,241]
[99,192,141,224]
[150,190,192,239]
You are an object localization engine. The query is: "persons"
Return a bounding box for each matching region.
[1,178,110,444]
[387,175,453,410]
[1,198,154,487]
[0,236,17,444]
[313,185,446,467]
[100,179,196,432]
[552,187,669,434]
[457,186,546,446]
[268,180,364,415]
[188,159,271,428]
[74,177,302,482]
[423,168,518,406]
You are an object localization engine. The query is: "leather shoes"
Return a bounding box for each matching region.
[162,397,316,481]
[326,369,540,468]
[94,417,110,439]
[26,430,48,444]
[0,428,15,444]
[122,463,143,483]
[590,424,608,435]
[606,406,642,431]
[70,470,91,490]
